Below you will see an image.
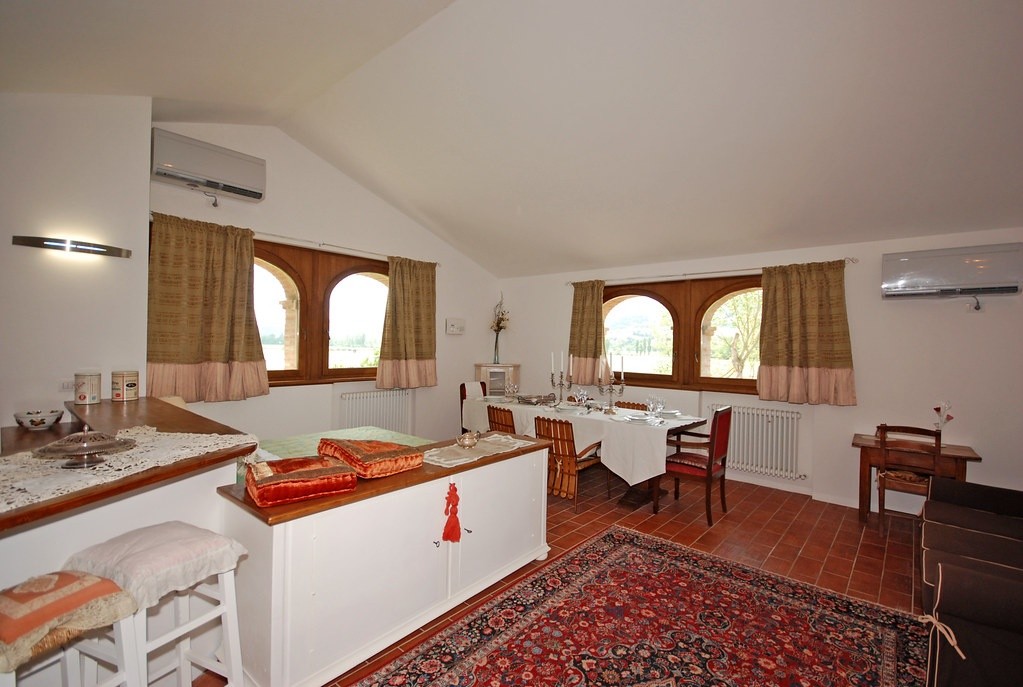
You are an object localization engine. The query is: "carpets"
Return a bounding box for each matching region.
[348,523,929,687]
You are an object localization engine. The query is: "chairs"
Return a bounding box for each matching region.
[487,405,516,435]
[653,406,733,527]
[615,401,649,411]
[534,415,612,515]
[877,423,942,538]
[459,381,487,434]
[567,396,595,402]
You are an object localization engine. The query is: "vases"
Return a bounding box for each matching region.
[493,332,500,364]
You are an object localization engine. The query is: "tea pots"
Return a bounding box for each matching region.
[456,430,481,449]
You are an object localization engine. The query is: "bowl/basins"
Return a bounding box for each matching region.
[13,409,64,430]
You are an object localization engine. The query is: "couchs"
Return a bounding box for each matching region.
[917,475,1023,616]
[925,559,1023,687]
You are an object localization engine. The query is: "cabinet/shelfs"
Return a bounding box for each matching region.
[474,362,521,396]
[217,433,554,687]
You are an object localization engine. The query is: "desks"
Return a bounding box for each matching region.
[461,395,708,515]
[236,425,436,484]
[851,433,982,523]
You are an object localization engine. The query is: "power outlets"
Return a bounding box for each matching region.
[205,198,224,210]
[965,303,986,313]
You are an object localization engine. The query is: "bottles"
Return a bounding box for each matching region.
[74,372,102,404]
[111,371,139,402]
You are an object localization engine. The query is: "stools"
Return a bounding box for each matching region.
[61,519,248,687]
[0,569,140,687]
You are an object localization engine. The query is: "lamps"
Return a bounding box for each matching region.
[11,234,133,260]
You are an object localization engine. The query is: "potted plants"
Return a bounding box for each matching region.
[150,127,267,205]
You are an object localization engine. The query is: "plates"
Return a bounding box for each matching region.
[556,405,578,410]
[627,415,649,421]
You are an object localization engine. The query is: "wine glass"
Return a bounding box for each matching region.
[504,383,518,396]
[571,388,587,405]
[647,393,657,414]
[655,397,665,419]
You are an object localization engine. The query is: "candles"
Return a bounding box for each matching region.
[560,351,563,371]
[550,351,554,372]
[598,355,602,378]
[609,352,613,376]
[569,354,573,376]
[621,356,624,380]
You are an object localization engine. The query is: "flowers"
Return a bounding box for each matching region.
[933,405,953,425]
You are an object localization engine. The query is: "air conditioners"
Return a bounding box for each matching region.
[880,241,1023,301]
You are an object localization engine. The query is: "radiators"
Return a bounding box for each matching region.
[710,403,809,481]
[339,388,412,434]
